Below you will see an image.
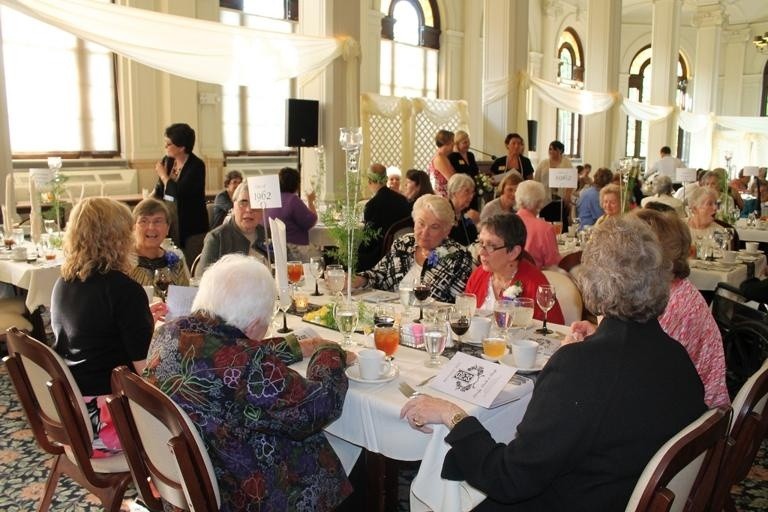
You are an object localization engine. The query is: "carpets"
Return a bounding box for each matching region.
[724,438,768,512]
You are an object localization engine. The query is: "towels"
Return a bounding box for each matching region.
[3,172,17,240]
[29,170,41,245]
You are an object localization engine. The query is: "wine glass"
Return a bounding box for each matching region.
[536,285,557,334]
[154,268,172,302]
[323,201,348,224]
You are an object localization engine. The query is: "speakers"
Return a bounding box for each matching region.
[286,98,319,147]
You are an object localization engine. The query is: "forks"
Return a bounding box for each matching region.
[399,381,435,399]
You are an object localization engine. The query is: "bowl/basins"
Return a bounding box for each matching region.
[29,169,52,184]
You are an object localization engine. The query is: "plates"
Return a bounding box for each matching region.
[345,364,400,384]
[716,260,743,266]
[497,355,550,374]
[739,249,765,254]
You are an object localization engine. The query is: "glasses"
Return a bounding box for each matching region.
[473,241,509,254]
[238,201,250,210]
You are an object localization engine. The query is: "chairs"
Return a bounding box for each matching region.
[623,404,735,512]
[3,326,133,512]
[729,356,768,484]
[0,191,767,339]
[107,365,223,511]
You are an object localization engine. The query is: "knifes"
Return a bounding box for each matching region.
[416,375,437,386]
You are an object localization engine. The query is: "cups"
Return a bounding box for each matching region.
[143,287,153,305]
[546,209,759,262]
[48,156,63,182]
[189,277,201,288]
[4,220,66,264]
[266,257,534,368]
[510,339,537,368]
[357,348,392,380]
